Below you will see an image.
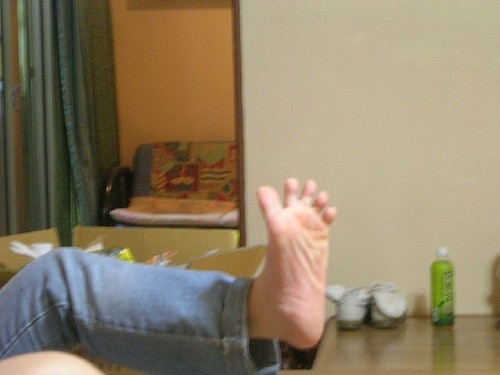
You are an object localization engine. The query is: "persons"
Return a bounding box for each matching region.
[0,175,336,374]
[1,352,106,375]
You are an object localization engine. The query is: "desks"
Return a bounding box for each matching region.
[311,312,499,375]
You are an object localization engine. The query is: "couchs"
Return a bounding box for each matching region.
[102,140,239,229]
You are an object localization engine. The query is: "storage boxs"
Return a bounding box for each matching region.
[0,223,268,375]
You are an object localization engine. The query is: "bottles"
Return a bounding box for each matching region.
[431,244,454,327]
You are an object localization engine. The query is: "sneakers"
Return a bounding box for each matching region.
[326,283,405,330]
